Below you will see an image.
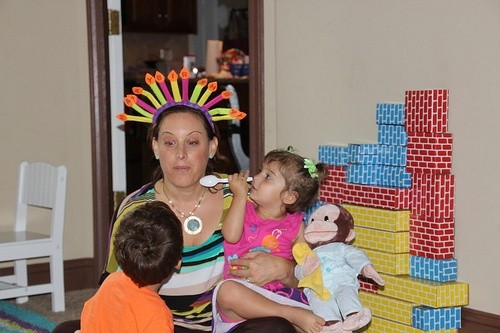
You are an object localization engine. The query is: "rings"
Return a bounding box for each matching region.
[246,278,250,283]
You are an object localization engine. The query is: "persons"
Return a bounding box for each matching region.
[79,201,184,333]
[212,150,327,333]
[99,68,301,333]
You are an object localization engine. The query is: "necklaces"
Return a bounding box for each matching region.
[162,181,206,235]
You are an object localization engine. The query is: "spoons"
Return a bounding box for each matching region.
[199,175,253,187]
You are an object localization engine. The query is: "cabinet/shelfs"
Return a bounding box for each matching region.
[124,85,249,178]
[121,0,199,35]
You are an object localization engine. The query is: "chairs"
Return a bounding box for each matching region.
[210,85,251,174]
[0,162,68,312]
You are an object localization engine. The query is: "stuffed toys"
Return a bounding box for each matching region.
[292,204,384,333]
